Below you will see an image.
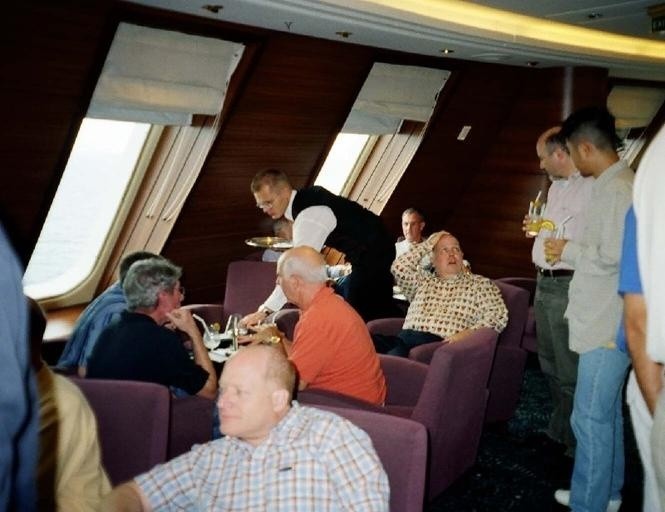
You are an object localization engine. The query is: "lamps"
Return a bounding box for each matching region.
[644,5,665,35]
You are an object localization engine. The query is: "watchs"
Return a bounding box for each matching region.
[265,336,282,345]
[258,306,271,316]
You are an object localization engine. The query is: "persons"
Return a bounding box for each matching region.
[23,290,110,512]
[99,345,390,512]
[554,104,634,512]
[616,205,664,505]
[247,246,387,407]
[390,207,434,299]
[236,166,397,319]
[56,250,161,377]
[390,231,510,358]
[522,125,582,445]
[89,259,217,402]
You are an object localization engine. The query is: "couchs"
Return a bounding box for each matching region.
[295,327,499,498]
[294,404,427,512]
[500,278,538,355]
[178,260,277,346]
[63,378,169,489]
[167,395,214,461]
[367,281,529,428]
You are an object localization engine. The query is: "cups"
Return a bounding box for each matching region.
[527,214,541,234]
[203,327,220,353]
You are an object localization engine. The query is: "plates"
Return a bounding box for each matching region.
[245,237,292,249]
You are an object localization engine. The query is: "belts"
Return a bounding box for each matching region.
[535,265,574,277]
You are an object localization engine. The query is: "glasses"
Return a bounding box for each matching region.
[256,193,279,208]
[165,286,185,297]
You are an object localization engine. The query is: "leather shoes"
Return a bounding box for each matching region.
[556,489,621,511]
